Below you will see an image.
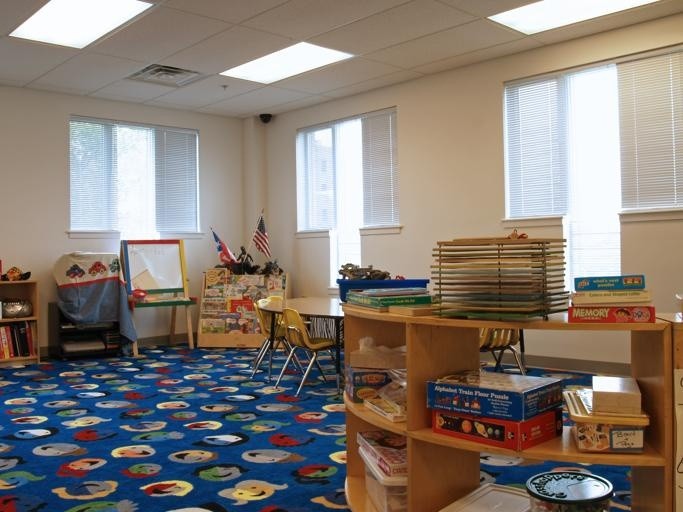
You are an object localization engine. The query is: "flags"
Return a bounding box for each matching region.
[251,209,273,259]
[207,225,239,266]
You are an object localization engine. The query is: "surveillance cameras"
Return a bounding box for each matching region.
[259,114,272,122]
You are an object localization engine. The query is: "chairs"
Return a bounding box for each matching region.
[479,326,525,376]
[246,293,344,399]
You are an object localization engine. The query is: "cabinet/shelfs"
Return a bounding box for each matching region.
[0,280,40,365]
[197,270,291,351]
[337,300,673,512]
[47,301,131,361]
[653,312,683,512]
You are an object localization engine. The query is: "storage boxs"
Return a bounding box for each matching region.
[562,387,650,454]
[438,482,533,512]
[358,446,408,512]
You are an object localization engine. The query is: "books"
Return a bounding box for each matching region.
[59,328,123,353]
[362,397,408,423]
[568,273,655,323]
[0,320,38,361]
[200,267,288,334]
[590,373,642,414]
[575,388,648,418]
[341,282,449,317]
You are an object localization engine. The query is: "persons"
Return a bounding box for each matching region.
[375,434,408,451]
[236,245,253,265]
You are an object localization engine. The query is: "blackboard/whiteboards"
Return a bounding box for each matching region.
[120,239,189,295]
